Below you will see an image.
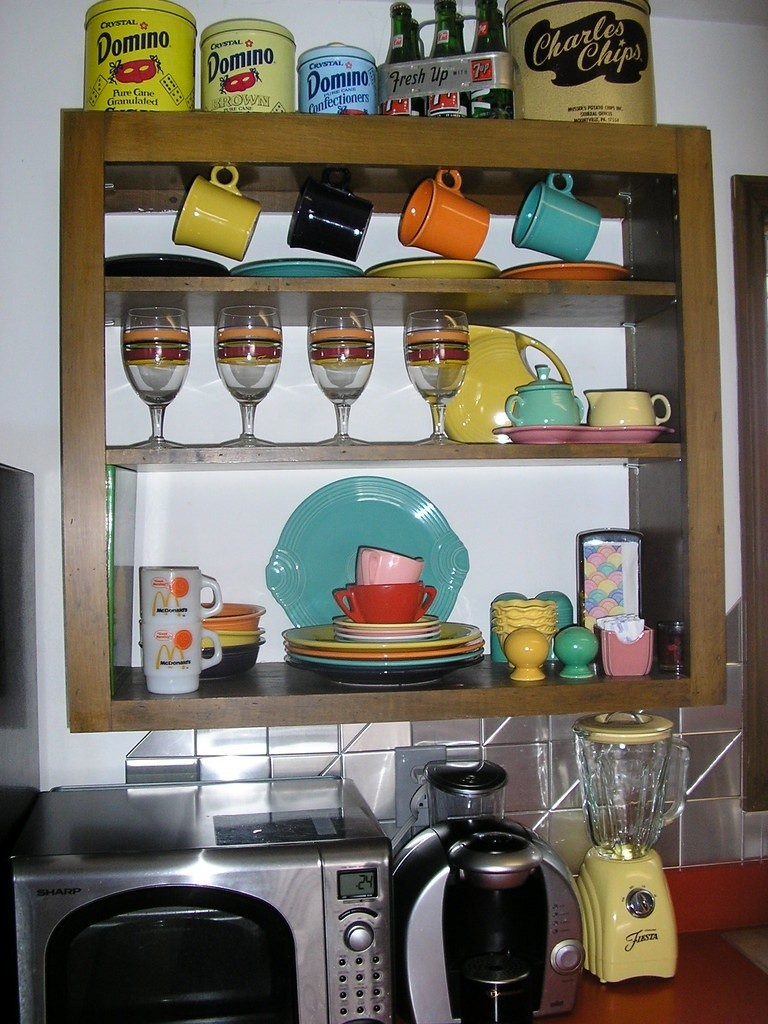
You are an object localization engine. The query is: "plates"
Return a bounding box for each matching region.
[492,425,675,444]
[499,262,630,281]
[283,614,486,686]
[229,261,365,277]
[105,253,229,277]
[265,475,470,628]
[365,257,501,279]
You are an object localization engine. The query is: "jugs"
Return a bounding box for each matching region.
[505,364,585,426]
[444,324,573,443]
[584,389,672,426]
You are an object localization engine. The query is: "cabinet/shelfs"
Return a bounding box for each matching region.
[55,109,723,730]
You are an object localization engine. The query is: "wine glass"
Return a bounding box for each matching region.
[120,305,192,448]
[306,306,375,446]
[214,305,283,449]
[402,309,469,445]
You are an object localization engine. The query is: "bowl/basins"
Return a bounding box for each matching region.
[199,603,267,681]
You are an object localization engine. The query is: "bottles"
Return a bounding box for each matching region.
[381,0,515,121]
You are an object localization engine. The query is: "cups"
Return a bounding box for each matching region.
[332,582,437,623]
[398,169,490,260]
[286,167,374,262]
[355,546,424,584]
[138,565,223,624]
[173,163,262,262]
[656,621,687,673]
[140,622,223,695]
[511,172,601,262]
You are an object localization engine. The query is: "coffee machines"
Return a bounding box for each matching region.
[388,756,586,1024]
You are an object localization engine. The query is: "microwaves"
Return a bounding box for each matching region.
[10,773,396,1024]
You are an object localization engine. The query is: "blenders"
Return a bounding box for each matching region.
[570,710,691,982]
